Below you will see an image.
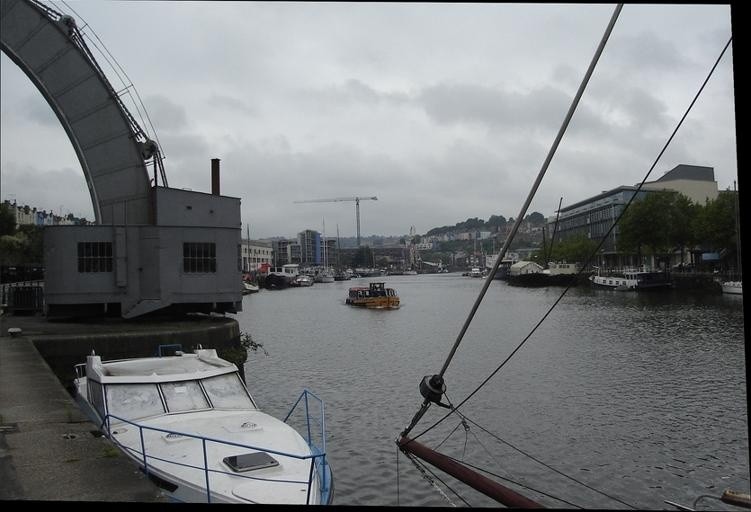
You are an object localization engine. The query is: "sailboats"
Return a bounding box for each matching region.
[715,179,744,294]
[396,0,750,511]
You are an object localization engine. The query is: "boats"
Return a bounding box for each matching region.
[240,279,260,293]
[590,283,640,300]
[402,267,418,274]
[471,268,482,276]
[294,214,387,287]
[71,342,335,505]
[346,282,400,308]
[589,264,641,289]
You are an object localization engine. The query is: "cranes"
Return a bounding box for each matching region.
[293,195,379,246]
